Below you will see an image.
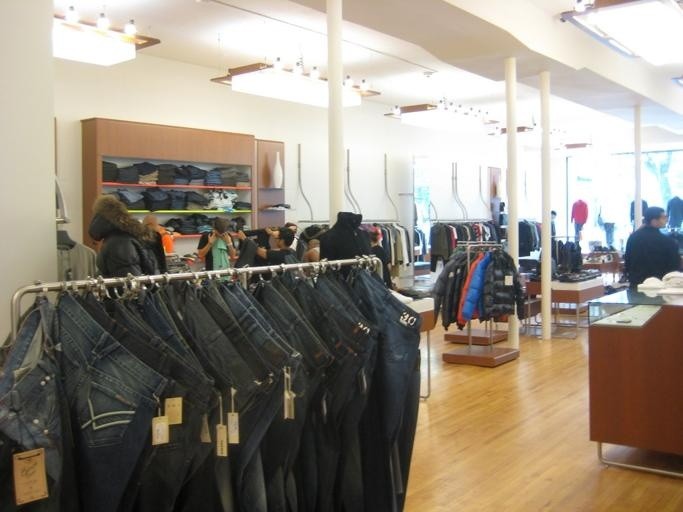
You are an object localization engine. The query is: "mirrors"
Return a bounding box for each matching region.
[413,156,431,276]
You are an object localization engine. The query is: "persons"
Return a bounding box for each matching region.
[624,207,681,288]
[196,219,394,291]
[141,215,168,275]
[550,210,557,240]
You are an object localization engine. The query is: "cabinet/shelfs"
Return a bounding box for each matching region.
[81,118,257,256]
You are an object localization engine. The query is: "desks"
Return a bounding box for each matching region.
[551,276,604,332]
